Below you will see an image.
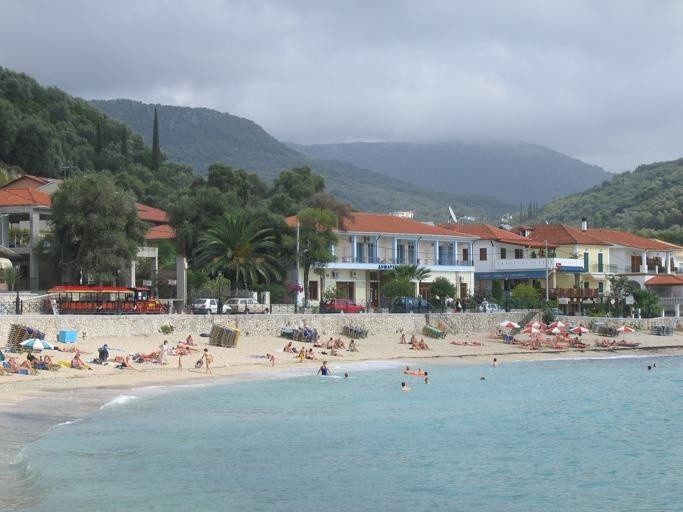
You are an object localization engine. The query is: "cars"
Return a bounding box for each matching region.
[192,297,269,315]
[479,302,506,312]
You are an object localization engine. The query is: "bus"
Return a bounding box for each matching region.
[45,284,170,315]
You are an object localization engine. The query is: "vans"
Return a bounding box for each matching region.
[318,298,366,314]
[390,297,437,313]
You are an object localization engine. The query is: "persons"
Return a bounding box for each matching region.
[1,347,93,374]
[454,297,489,313]
[401,366,430,390]
[495,330,640,350]
[401,323,484,349]
[267,329,359,377]
[98,343,159,370]
[158,335,214,374]
[493,358,498,366]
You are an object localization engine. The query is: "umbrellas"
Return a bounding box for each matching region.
[526,320,547,327]
[545,320,567,328]
[501,320,520,329]
